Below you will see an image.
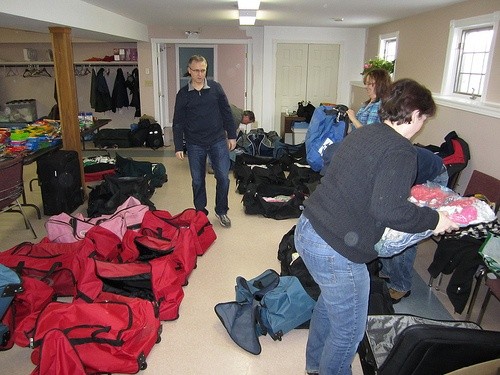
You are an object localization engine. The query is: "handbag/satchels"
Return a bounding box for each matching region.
[305,103,353,173]
[0,127,500,375]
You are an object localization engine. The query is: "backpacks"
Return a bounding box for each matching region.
[144,122,165,151]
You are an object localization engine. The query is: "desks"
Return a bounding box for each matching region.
[0,119,112,218]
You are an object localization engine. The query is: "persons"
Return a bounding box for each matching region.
[208,104,255,174]
[437,131,470,189]
[294,78,459,375]
[346,69,392,130]
[173,55,236,228]
[379,146,449,304]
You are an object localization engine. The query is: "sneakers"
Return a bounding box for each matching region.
[215,212,232,227]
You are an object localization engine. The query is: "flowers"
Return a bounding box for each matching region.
[362,57,394,75]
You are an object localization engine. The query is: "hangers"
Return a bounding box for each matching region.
[6,64,123,78]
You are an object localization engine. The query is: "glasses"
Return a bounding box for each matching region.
[188,66,208,73]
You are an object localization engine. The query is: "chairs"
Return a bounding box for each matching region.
[0,156,38,239]
[429,170,500,325]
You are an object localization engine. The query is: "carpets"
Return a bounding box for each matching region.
[393,267,455,320]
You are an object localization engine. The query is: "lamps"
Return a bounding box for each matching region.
[185,31,199,40]
